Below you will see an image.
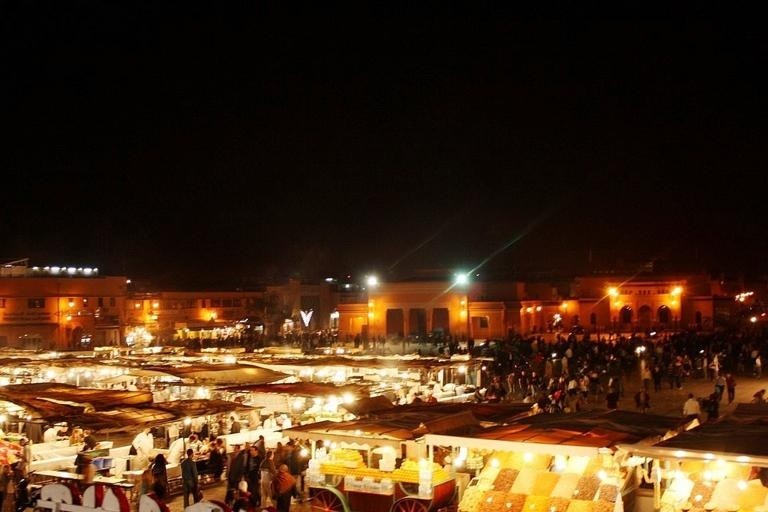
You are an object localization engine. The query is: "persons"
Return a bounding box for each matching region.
[0,325,767,512]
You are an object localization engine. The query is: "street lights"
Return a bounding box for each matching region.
[455,272,471,351]
